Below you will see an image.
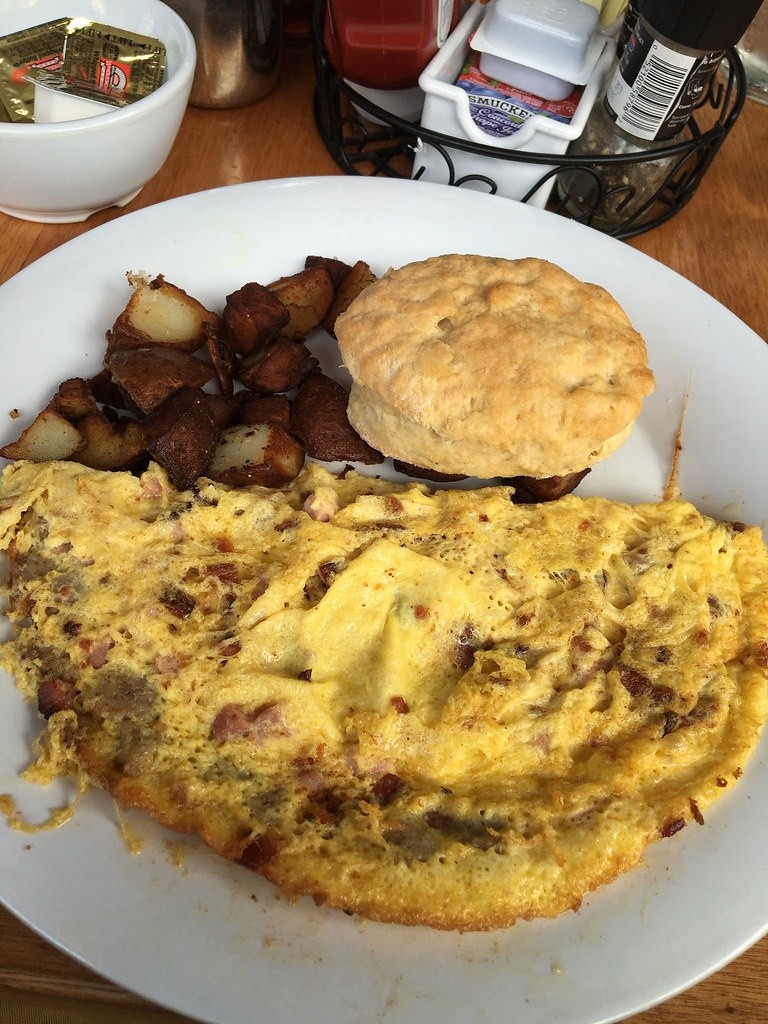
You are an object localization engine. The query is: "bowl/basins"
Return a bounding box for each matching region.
[162,0,318,111]
[0,3,199,225]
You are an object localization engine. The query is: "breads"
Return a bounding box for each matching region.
[331,256,656,482]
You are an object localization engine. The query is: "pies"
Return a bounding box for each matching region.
[0,458,768,937]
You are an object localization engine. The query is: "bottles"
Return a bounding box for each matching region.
[325,2,464,130]
[604,0,765,150]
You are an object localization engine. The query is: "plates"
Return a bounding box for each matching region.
[3,177,767,1024]
